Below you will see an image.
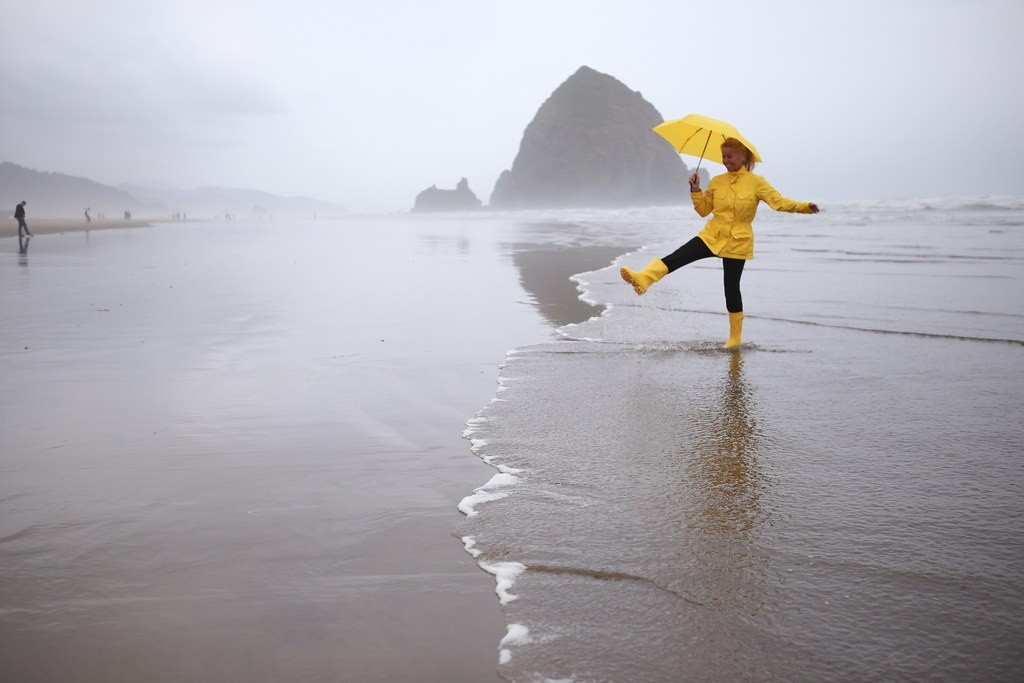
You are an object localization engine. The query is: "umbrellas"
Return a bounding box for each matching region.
[650,112,763,193]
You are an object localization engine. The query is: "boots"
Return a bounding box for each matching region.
[620,257,668,294]
[723,312,745,349]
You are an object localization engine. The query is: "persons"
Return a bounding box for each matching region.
[83,200,245,228]
[13,200,34,236]
[619,136,819,351]
